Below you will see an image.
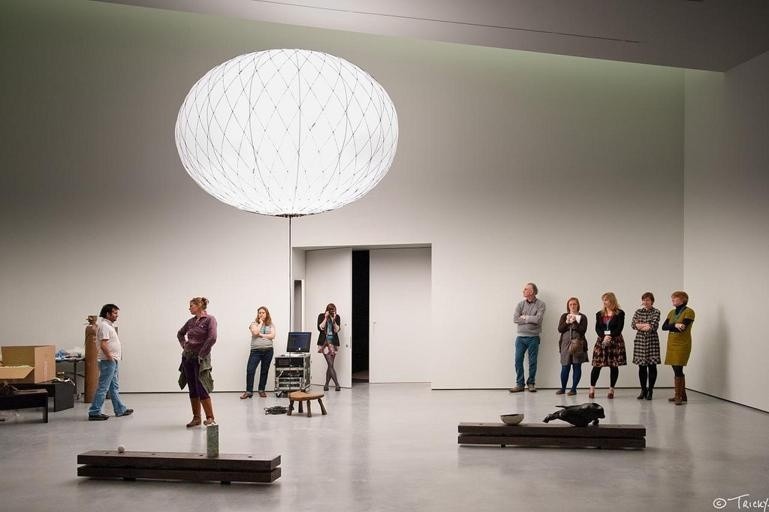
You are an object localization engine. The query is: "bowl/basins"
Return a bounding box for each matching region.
[499,413,524,425]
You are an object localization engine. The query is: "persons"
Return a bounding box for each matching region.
[89,304,133,420]
[510,284,545,393]
[239,307,275,398]
[317,304,340,391]
[631,293,661,400]
[178,297,217,427]
[589,292,627,399]
[556,298,588,395]
[662,291,695,405]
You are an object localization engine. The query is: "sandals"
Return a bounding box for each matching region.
[186,416,202,427]
[204,418,217,425]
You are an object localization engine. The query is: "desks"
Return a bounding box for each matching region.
[55,355,84,400]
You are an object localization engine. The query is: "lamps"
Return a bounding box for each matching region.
[173,49,399,218]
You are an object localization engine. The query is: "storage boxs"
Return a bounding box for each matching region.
[0,346,56,384]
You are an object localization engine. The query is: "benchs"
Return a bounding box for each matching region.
[0,389,47,423]
[7,383,73,412]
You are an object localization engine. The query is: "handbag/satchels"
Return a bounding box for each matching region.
[568,327,586,355]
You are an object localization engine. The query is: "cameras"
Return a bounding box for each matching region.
[327,310,334,324]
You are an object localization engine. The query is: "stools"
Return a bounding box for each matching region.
[286,391,327,417]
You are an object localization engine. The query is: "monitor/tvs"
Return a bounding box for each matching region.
[286,332,311,352]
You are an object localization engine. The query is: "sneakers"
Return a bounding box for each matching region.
[89,414,110,421]
[567,391,576,395]
[528,384,536,392]
[120,408,134,416]
[556,389,565,394]
[510,386,525,393]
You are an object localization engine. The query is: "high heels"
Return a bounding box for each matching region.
[607,388,614,399]
[240,392,253,399]
[258,390,266,397]
[647,390,653,400]
[637,389,648,399]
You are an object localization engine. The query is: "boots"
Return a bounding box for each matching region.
[669,390,687,402]
[675,377,685,405]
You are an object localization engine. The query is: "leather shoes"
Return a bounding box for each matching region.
[335,386,341,391]
[324,386,329,391]
[589,393,594,398]
